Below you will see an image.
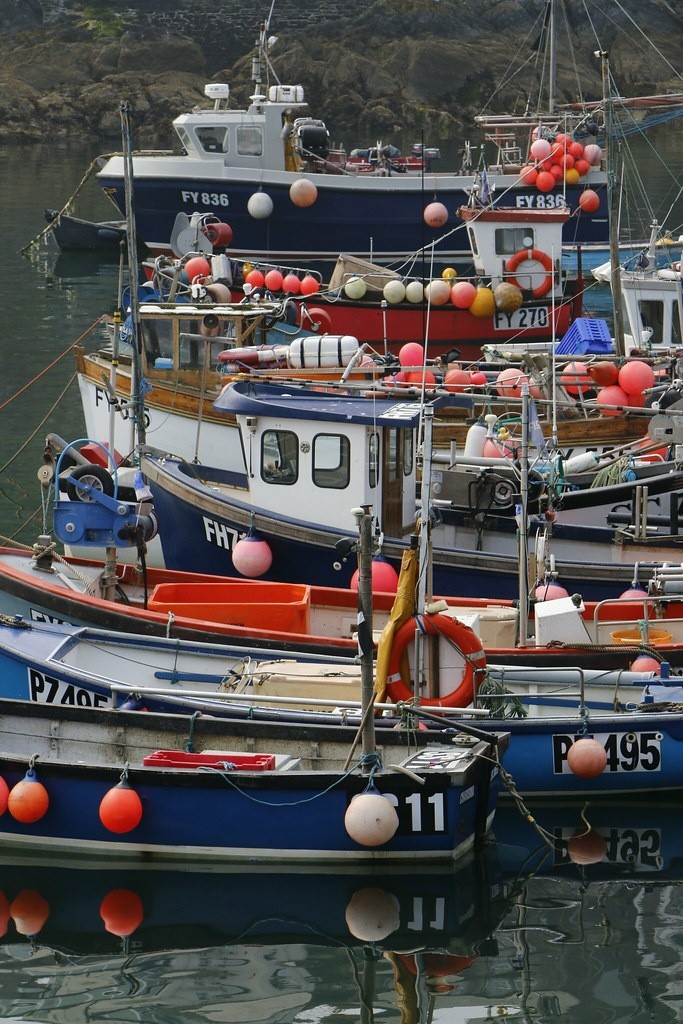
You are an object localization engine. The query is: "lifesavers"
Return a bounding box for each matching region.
[215,345,291,372]
[384,614,486,716]
[505,249,555,298]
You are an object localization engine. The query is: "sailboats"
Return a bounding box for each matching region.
[35,1,682,603]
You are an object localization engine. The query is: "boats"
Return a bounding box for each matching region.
[0,513,512,861]
[0,546,683,674]
[42,207,128,252]
[0,613,683,805]
